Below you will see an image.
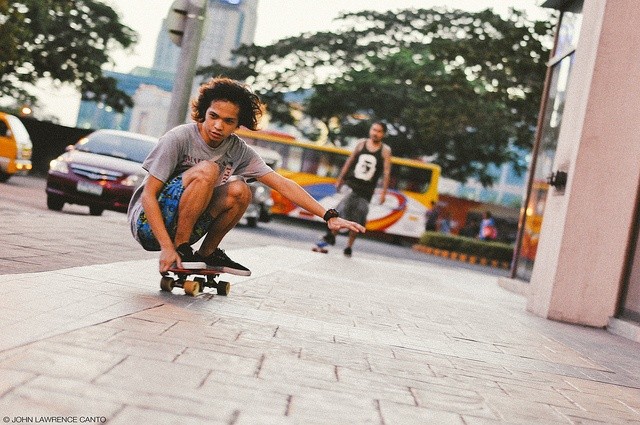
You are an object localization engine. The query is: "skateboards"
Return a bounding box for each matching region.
[159,268,230,297]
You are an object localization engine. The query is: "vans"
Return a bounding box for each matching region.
[0,109,34,184]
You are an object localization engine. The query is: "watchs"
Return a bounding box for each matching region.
[322,208,339,221]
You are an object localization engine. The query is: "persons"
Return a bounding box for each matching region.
[477,211,498,241]
[425,201,438,232]
[318,122,391,258]
[126,74,365,277]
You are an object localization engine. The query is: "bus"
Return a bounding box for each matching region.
[226,129,439,244]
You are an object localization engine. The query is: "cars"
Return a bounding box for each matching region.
[233,144,281,229]
[46,126,158,217]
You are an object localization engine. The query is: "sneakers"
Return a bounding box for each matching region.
[171,243,206,269]
[344,247,351,254]
[315,235,335,245]
[194,248,251,276]
